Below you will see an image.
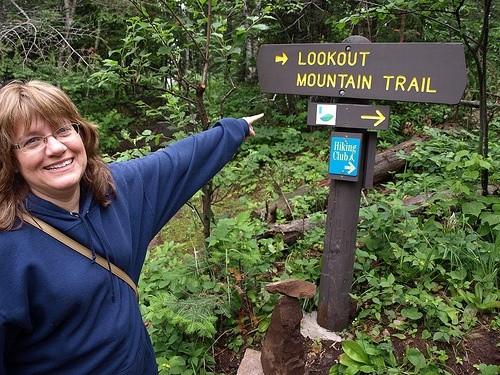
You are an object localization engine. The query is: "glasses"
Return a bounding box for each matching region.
[11,123,79,153]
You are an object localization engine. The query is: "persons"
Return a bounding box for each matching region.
[0,79,266,375]
